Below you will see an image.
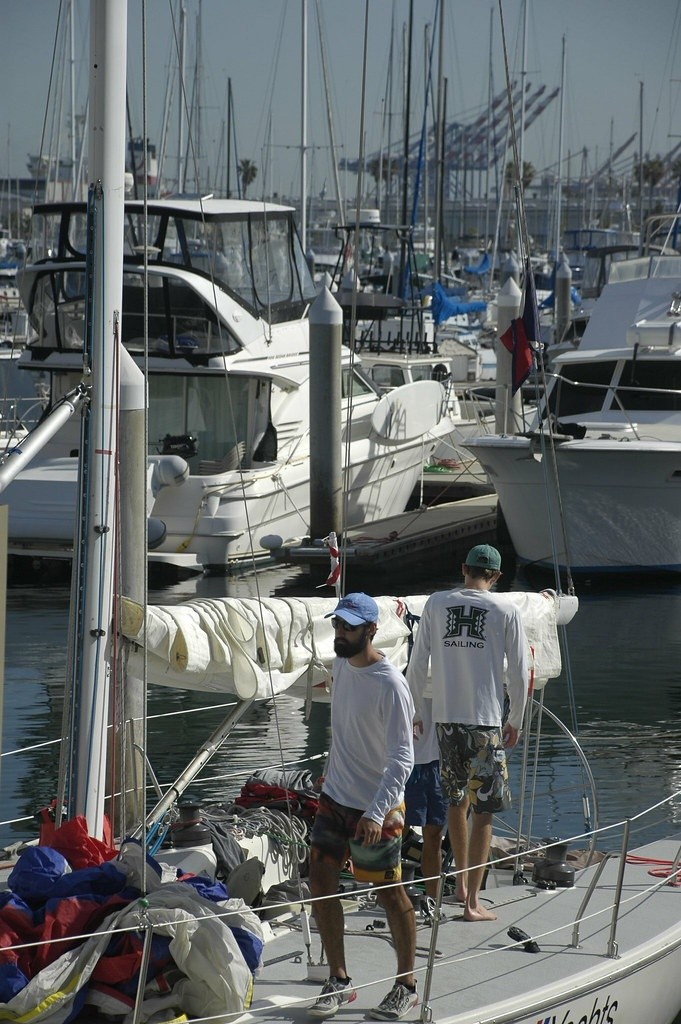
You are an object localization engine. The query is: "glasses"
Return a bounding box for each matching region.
[331,618,370,632]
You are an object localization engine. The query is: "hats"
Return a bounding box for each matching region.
[466,544,501,570]
[324,592,378,625]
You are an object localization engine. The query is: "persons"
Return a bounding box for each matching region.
[307,594,419,1020]
[405,545,527,921]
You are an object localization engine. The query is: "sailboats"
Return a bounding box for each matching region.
[0,0,681,1024]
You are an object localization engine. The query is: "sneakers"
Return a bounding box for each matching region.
[306,977,357,1016]
[369,978,419,1021]
